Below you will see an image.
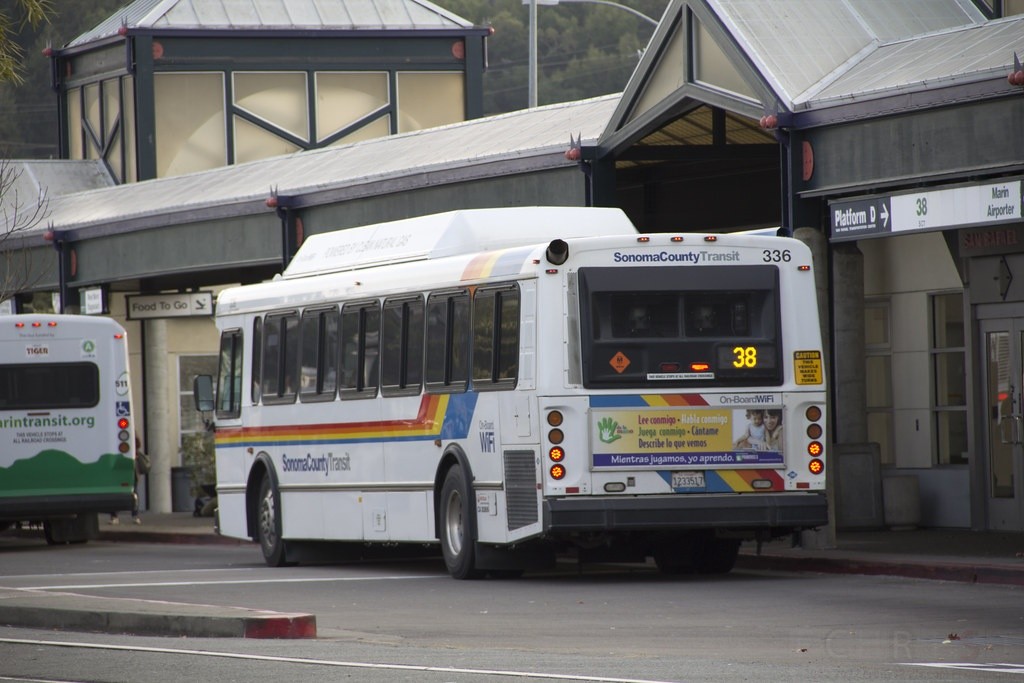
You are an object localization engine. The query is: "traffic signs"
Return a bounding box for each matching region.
[829,176,1024,243]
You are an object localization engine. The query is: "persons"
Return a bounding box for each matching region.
[734,409,783,451]
[108,462,141,524]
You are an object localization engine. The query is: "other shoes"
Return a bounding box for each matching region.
[132,518,141,525]
[108,517,119,525]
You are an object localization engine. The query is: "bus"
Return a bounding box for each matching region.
[1,313,138,550]
[191,205,831,582]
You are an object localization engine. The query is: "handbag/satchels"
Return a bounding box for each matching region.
[137,451,152,474]
